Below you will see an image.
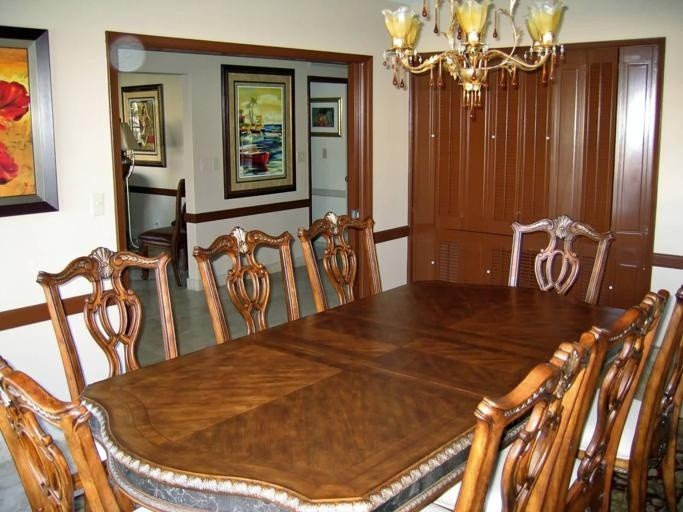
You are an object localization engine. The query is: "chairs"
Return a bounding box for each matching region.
[0,357,151,511]
[139,179,187,287]
[298,212,383,314]
[37,247,177,404]
[193,225,300,345]
[508,214,614,306]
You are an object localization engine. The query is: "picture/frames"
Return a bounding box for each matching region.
[221,64,294,199]
[121,83,166,166]
[0,25,59,216]
[310,97,342,137]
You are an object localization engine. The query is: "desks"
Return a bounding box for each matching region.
[80,279,627,512]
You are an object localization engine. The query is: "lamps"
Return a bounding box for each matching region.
[119,123,141,249]
[381,0,566,106]
